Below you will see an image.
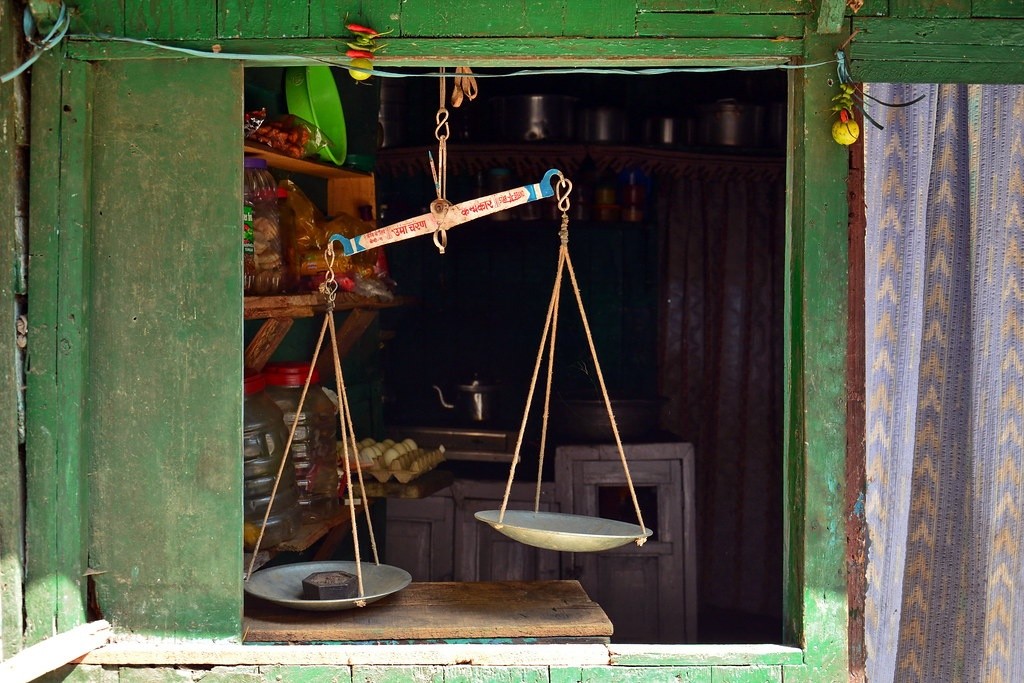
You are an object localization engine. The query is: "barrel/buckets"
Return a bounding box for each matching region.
[243,364,340,553]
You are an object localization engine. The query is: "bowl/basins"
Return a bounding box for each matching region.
[554,399,656,442]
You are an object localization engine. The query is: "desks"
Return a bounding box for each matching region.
[238,577,613,643]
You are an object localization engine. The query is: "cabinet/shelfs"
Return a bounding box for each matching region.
[553,441,697,643]
[370,474,562,585]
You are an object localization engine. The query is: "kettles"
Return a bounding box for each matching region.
[432,372,510,425]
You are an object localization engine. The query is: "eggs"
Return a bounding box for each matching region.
[335,436,418,466]
